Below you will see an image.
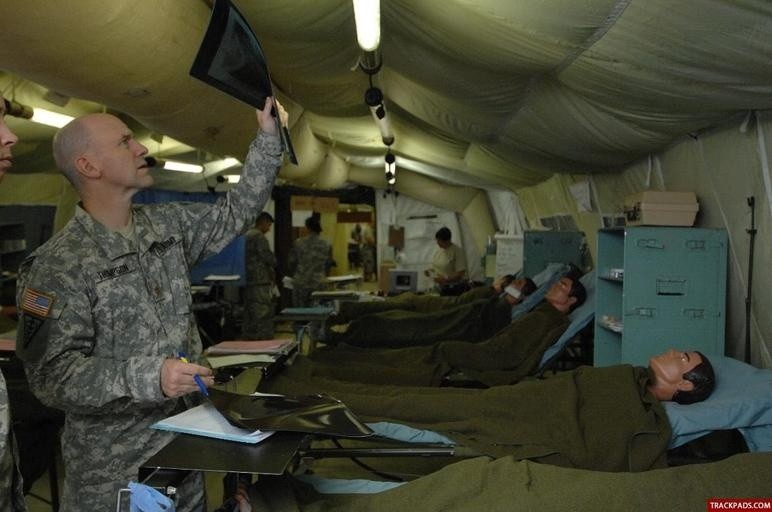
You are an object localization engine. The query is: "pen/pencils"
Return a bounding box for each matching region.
[178,352,209,397]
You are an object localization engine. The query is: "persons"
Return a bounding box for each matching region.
[234,480,252,512]
[646,349,716,405]
[504,277,538,306]
[244,211,279,339]
[0,90,27,511]
[545,275,587,318]
[423,227,467,295]
[287,216,331,308]
[494,274,515,294]
[15,96,294,512]
[350,222,377,282]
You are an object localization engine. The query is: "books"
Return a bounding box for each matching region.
[148,403,278,445]
[204,338,296,369]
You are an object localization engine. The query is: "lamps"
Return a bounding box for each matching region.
[144,156,241,184]
[352,0,396,184]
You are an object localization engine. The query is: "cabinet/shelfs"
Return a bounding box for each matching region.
[494,229,584,280]
[593,227,728,368]
